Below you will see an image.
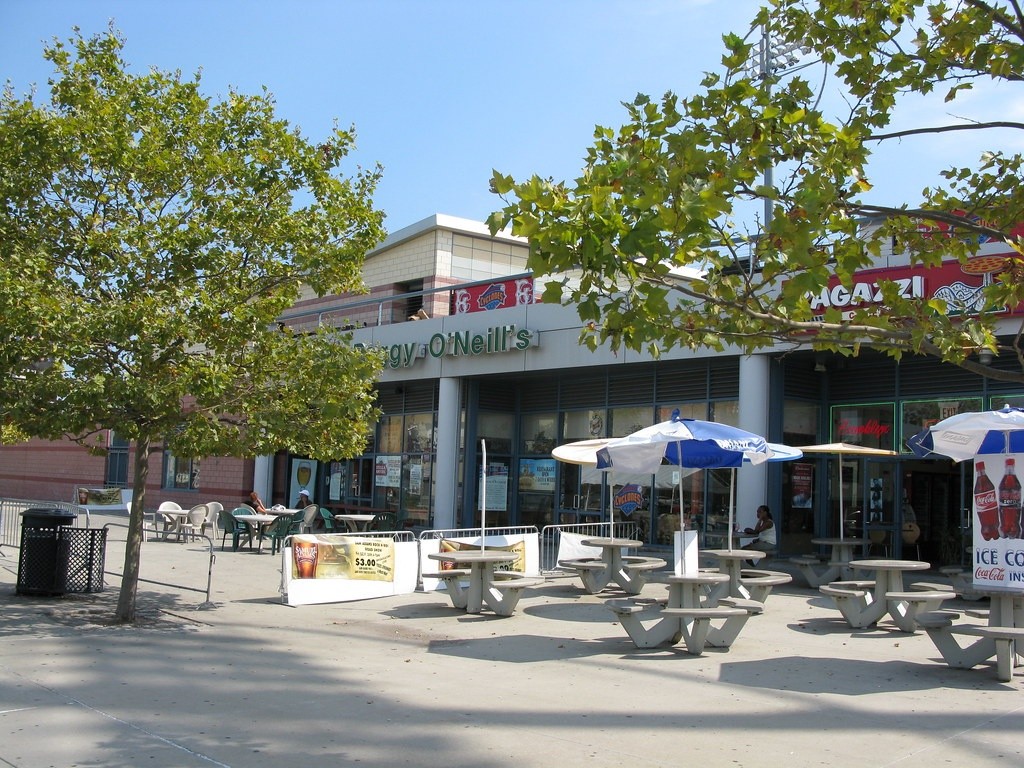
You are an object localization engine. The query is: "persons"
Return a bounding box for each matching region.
[741,505,777,567]
[244,492,270,540]
[295,490,312,510]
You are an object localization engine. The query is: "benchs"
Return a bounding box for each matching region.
[75,487,133,515]
[558,556,1024,682]
[422,569,545,616]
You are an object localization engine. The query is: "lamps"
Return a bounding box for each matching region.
[977,349,995,365]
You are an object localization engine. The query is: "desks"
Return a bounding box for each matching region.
[428,550,519,614]
[699,549,766,615]
[639,572,730,655]
[265,509,303,517]
[156,510,190,543]
[953,584,1024,681]
[335,514,386,532]
[581,538,643,594]
[848,560,931,633]
[810,538,872,588]
[233,514,279,552]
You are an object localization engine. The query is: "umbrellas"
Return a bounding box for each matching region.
[905,404,1024,463]
[596,409,774,530]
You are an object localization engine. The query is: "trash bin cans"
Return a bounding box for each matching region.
[15,507,79,598]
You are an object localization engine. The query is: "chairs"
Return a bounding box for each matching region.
[867,521,891,558]
[901,522,920,561]
[320,507,346,533]
[369,509,409,542]
[127,502,158,542]
[159,501,319,555]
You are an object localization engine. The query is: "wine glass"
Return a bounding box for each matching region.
[296,462,312,494]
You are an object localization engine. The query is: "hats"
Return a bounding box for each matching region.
[299,489,310,497]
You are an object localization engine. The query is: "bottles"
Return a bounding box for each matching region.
[974,461,999,541]
[1000,459,1022,539]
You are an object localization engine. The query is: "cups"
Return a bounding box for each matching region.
[294,542,318,580]
[80,492,88,505]
[441,549,458,572]
